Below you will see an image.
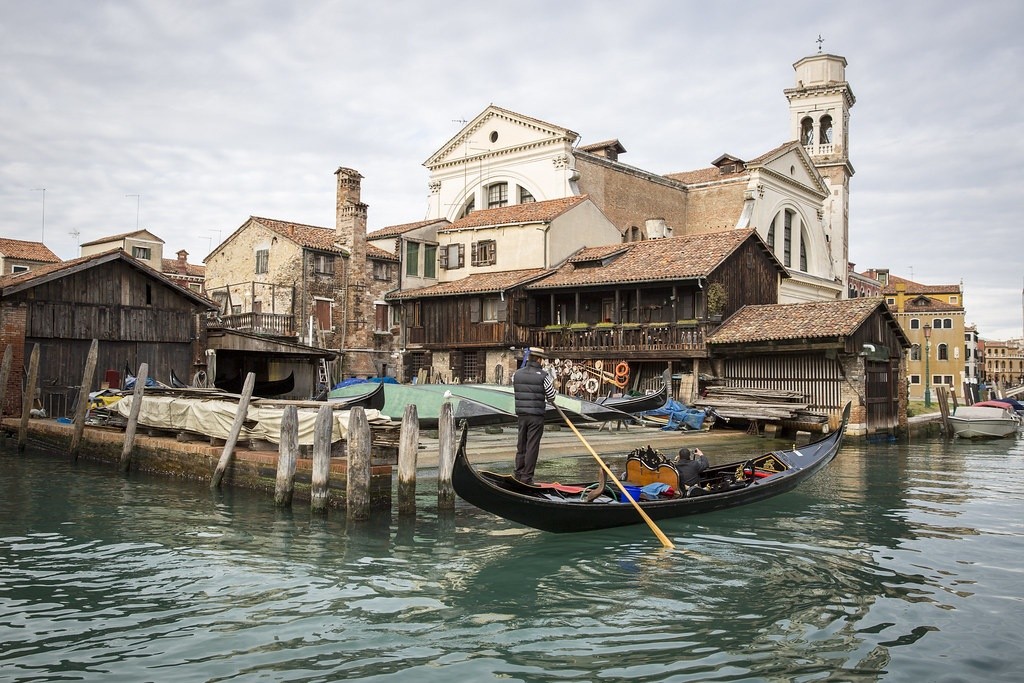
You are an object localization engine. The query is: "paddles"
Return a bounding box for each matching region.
[553,400,677,549]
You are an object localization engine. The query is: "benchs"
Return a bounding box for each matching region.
[625,445,687,499]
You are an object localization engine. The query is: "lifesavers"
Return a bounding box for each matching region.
[584,378,599,394]
[614,363,630,386]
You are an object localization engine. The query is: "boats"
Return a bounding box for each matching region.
[452,402,851,532]
[947,398,1023,440]
[319,380,386,413]
[513,382,666,424]
[329,382,640,429]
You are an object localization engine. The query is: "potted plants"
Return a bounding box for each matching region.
[707,282,729,321]
[545,319,699,332]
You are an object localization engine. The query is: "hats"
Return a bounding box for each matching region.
[529,347,550,358]
[595,360,602,370]
[554,358,589,394]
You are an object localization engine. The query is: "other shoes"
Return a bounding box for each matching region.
[529,484,541,487]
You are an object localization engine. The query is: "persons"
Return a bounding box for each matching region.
[675,448,709,497]
[511,347,556,487]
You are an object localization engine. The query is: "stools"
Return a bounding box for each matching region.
[747,418,760,435]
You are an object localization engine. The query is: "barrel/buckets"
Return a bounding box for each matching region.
[621,486,643,503]
